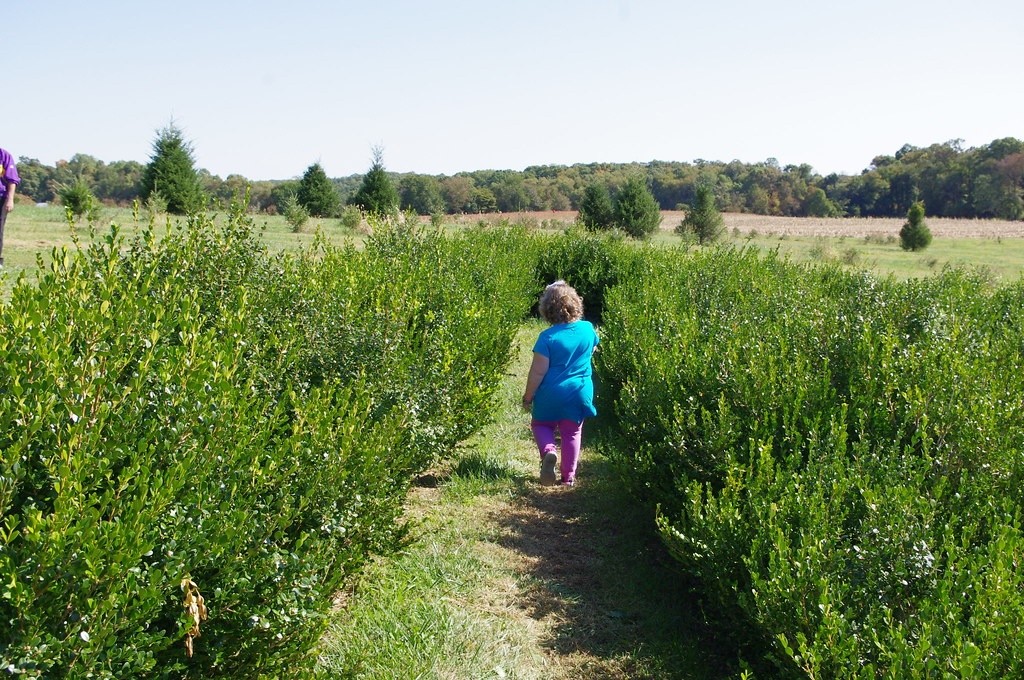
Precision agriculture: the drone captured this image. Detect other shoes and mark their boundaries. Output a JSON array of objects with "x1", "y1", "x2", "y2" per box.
[
  {"x1": 560, "y1": 477, "x2": 573, "y2": 487},
  {"x1": 541, "y1": 451, "x2": 556, "y2": 485}
]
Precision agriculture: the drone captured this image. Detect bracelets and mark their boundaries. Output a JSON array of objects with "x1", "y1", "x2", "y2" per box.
[{"x1": 522, "y1": 394, "x2": 531, "y2": 404}]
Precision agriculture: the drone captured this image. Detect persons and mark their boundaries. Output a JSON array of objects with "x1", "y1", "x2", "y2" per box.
[
  {"x1": 0, "y1": 147, "x2": 21, "y2": 264},
  {"x1": 521, "y1": 281, "x2": 600, "y2": 486}
]
